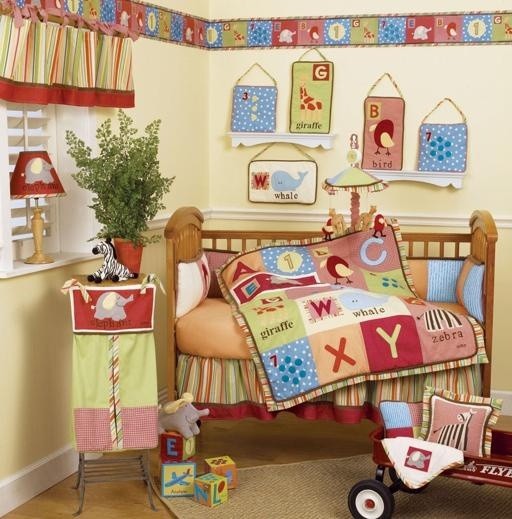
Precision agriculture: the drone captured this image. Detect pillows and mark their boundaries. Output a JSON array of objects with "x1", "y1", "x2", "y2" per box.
[
  {"x1": 454, "y1": 255, "x2": 485, "y2": 330},
  {"x1": 202, "y1": 248, "x2": 240, "y2": 298},
  {"x1": 407, "y1": 255, "x2": 466, "y2": 303},
  {"x1": 419, "y1": 383, "x2": 502, "y2": 457},
  {"x1": 378, "y1": 400, "x2": 423, "y2": 438},
  {"x1": 174, "y1": 250, "x2": 212, "y2": 319}
]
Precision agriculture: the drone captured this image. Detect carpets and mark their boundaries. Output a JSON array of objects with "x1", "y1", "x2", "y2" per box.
[{"x1": 145, "y1": 452, "x2": 511, "y2": 518}]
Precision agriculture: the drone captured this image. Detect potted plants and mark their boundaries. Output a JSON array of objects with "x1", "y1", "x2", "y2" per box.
[{"x1": 63, "y1": 107, "x2": 175, "y2": 275}]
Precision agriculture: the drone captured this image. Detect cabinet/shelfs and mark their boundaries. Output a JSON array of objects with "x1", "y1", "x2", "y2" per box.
[{"x1": 69, "y1": 273, "x2": 159, "y2": 515}]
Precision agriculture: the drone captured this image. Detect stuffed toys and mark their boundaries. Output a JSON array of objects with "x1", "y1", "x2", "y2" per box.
[
  {"x1": 156, "y1": 391, "x2": 213, "y2": 441},
  {"x1": 89, "y1": 238, "x2": 139, "y2": 283}
]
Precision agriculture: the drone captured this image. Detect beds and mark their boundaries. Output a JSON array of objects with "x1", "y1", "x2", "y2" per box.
[{"x1": 159, "y1": 205, "x2": 498, "y2": 424}]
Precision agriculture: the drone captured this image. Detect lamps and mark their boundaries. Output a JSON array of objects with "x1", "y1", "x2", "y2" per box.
[{"x1": 9, "y1": 151, "x2": 66, "y2": 264}]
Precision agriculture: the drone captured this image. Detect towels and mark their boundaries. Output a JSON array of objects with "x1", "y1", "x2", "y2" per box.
[{"x1": 381, "y1": 437, "x2": 465, "y2": 490}]
